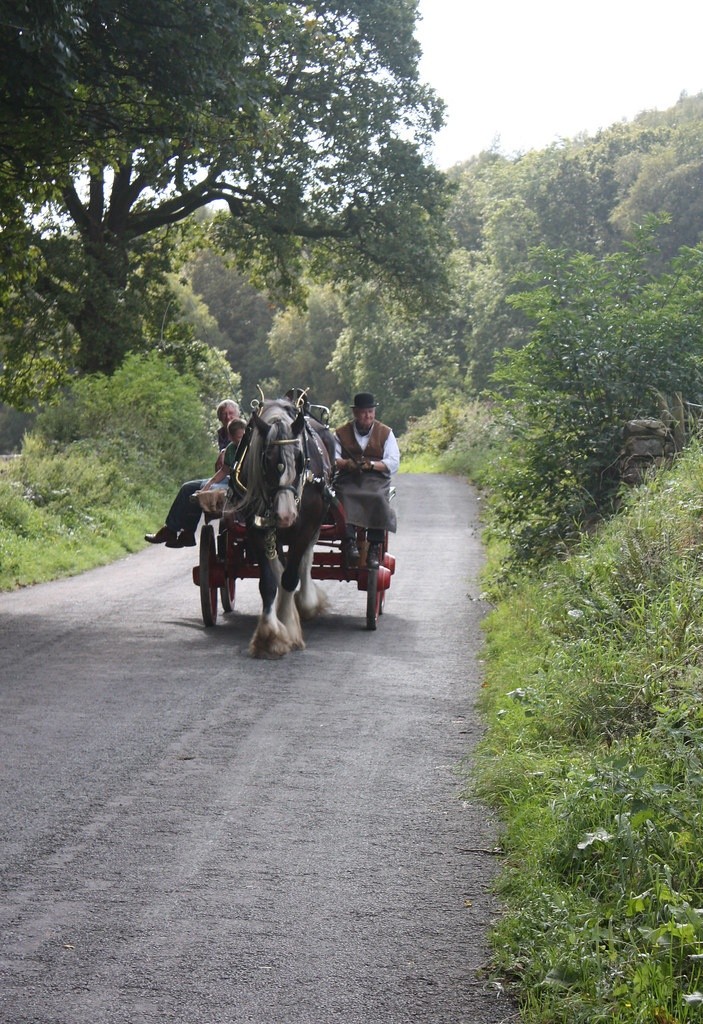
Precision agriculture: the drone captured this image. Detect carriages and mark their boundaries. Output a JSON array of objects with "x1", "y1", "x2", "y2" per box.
[{"x1": 191, "y1": 381, "x2": 397, "y2": 659}]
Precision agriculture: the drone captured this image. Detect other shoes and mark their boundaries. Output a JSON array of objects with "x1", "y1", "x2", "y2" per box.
[
  {"x1": 145, "y1": 526, "x2": 177, "y2": 544},
  {"x1": 165, "y1": 530, "x2": 196, "y2": 548},
  {"x1": 366, "y1": 547, "x2": 380, "y2": 568},
  {"x1": 340, "y1": 536, "x2": 360, "y2": 558}
]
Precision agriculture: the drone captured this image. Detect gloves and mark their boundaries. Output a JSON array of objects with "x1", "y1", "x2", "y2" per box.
[{"x1": 347, "y1": 458, "x2": 372, "y2": 474}]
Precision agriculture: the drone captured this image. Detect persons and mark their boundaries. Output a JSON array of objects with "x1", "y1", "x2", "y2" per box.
[
  {"x1": 145, "y1": 399, "x2": 247, "y2": 548},
  {"x1": 333, "y1": 392, "x2": 399, "y2": 570}
]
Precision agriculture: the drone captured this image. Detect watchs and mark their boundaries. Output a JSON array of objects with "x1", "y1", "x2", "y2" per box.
[{"x1": 370, "y1": 461, "x2": 375, "y2": 471}]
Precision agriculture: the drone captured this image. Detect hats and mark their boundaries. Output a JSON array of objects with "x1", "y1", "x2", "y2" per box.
[
  {"x1": 284, "y1": 387, "x2": 307, "y2": 408},
  {"x1": 350, "y1": 393, "x2": 379, "y2": 408}
]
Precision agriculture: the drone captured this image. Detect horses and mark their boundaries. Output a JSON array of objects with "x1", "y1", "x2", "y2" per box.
[{"x1": 222, "y1": 388, "x2": 339, "y2": 661}]
